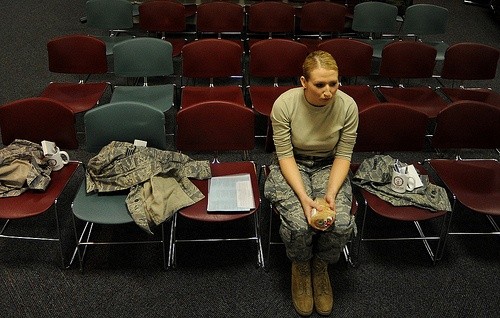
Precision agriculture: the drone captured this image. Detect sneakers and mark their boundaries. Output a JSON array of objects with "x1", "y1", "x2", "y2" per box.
[
  {"x1": 311, "y1": 261, "x2": 333, "y2": 316},
  {"x1": 290, "y1": 260, "x2": 313, "y2": 316}
]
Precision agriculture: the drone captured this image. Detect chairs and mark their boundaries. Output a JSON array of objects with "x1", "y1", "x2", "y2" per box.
[{"x1": 0, "y1": 0, "x2": 500, "y2": 269}]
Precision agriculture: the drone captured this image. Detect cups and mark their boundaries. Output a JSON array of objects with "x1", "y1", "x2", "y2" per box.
[
  {"x1": 391, "y1": 168, "x2": 415, "y2": 194},
  {"x1": 44, "y1": 146, "x2": 69, "y2": 171}
]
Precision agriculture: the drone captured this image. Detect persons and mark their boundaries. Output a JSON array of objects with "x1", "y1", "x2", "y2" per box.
[{"x1": 264, "y1": 51, "x2": 359, "y2": 315}]
